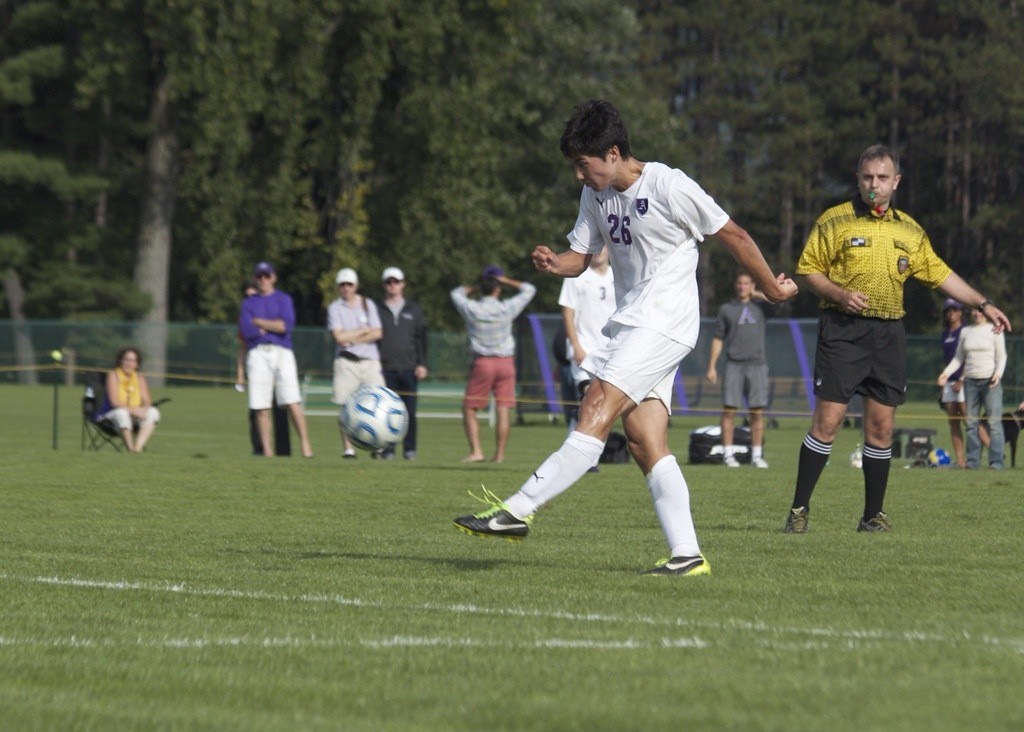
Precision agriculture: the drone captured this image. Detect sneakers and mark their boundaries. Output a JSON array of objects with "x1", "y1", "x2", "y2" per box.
[
  {"x1": 638, "y1": 550, "x2": 712, "y2": 580},
  {"x1": 452, "y1": 483, "x2": 535, "y2": 543},
  {"x1": 783, "y1": 504, "x2": 810, "y2": 534},
  {"x1": 856, "y1": 510, "x2": 895, "y2": 533}
]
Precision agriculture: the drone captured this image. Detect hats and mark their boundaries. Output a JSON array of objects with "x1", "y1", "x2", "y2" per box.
[
  {"x1": 255, "y1": 261, "x2": 272, "y2": 276},
  {"x1": 381, "y1": 266, "x2": 406, "y2": 281},
  {"x1": 242, "y1": 279, "x2": 256, "y2": 287},
  {"x1": 943, "y1": 297, "x2": 963, "y2": 312},
  {"x1": 480, "y1": 265, "x2": 504, "y2": 278},
  {"x1": 335, "y1": 267, "x2": 359, "y2": 286}
]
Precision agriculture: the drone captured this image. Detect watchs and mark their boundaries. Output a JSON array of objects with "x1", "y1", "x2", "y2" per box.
[{"x1": 977, "y1": 299, "x2": 994, "y2": 312}]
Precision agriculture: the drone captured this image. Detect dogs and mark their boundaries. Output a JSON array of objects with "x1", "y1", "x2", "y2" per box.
[{"x1": 980, "y1": 409, "x2": 1024, "y2": 467}]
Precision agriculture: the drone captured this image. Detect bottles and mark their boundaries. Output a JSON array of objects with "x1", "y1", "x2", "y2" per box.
[
  {"x1": 85, "y1": 388, "x2": 94, "y2": 398},
  {"x1": 853, "y1": 442, "x2": 863, "y2": 469}
]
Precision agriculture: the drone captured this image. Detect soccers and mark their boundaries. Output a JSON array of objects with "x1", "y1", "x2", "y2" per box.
[{"x1": 338, "y1": 385, "x2": 410, "y2": 454}]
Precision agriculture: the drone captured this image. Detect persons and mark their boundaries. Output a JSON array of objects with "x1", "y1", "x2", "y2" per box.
[
  {"x1": 453, "y1": 101, "x2": 798, "y2": 577},
  {"x1": 784, "y1": 146, "x2": 1012, "y2": 534},
  {"x1": 941, "y1": 298, "x2": 1006, "y2": 469},
  {"x1": 237, "y1": 242, "x2": 617, "y2": 475},
  {"x1": 707, "y1": 271, "x2": 777, "y2": 468},
  {"x1": 95, "y1": 346, "x2": 160, "y2": 453},
  {"x1": 938, "y1": 307, "x2": 1007, "y2": 469}
]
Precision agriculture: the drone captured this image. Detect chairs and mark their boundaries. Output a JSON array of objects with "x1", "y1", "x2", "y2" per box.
[{"x1": 80, "y1": 370, "x2": 138, "y2": 454}]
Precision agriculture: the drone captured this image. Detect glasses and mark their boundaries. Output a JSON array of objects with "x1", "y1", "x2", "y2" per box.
[
  {"x1": 339, "y1": 283, "x2": 353, "y2": 286},
  {"x1": 385, "y1": 280, "x2": 399, "y2": 284}
]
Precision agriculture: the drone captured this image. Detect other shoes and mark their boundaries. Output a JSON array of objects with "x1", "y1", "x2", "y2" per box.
[
  {"x1": 948, "y1": 454, "x2": 1006, "y2": 471},
  {"x1": 372, "y1": 450, "x2": 396, "y2": 460},
  {"x1": 750, "y1": 458, "x2": 768, "y2": 468},
  {"x1": 234, "y1": 384, "x2": 244, "y2": 392},
  {"x1": 462, "y1": 456, "x2": 503, "y2": 464},
  {"x1": 342, "y1": 449, "x2": 355, "y2": 457},
  {"x1": 404, "y1": 450, "x2": 416, "y2": 460},
  {"x1": 723, "y1": 456, "x2": 740, "y2": 468}
]
